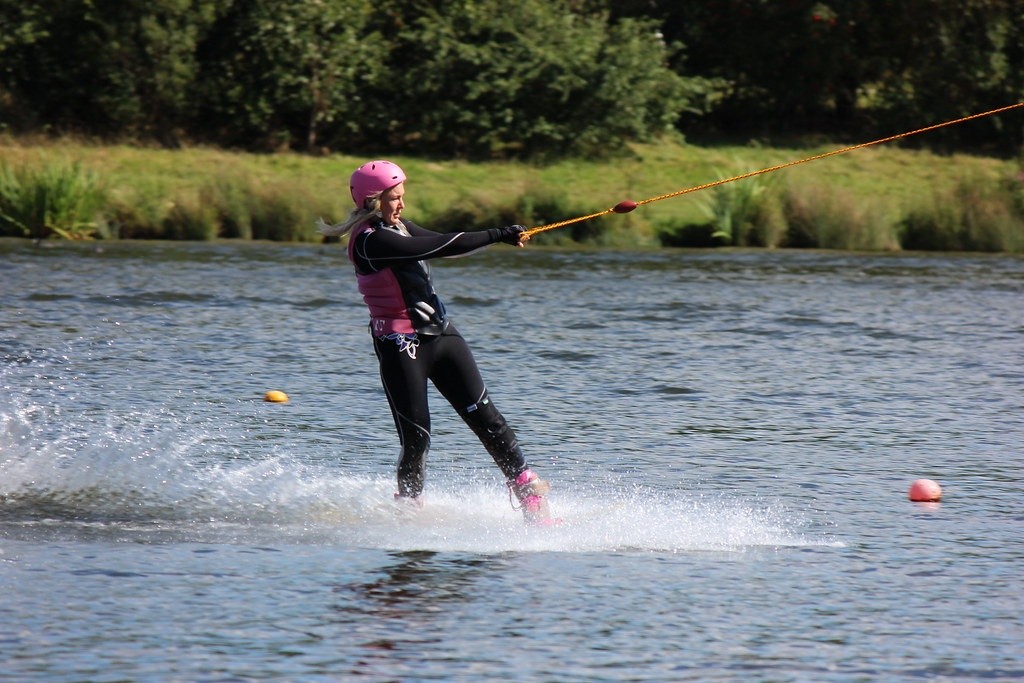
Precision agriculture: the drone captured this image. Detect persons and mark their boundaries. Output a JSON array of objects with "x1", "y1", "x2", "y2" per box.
[{"x1": 347, "y1": 160, "x2": 553, "y2": 529}]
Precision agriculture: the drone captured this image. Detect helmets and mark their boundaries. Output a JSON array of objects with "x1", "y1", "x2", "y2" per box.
[{"x1": 351, "y1": 160, "x2": 406, "y2": 209}]
